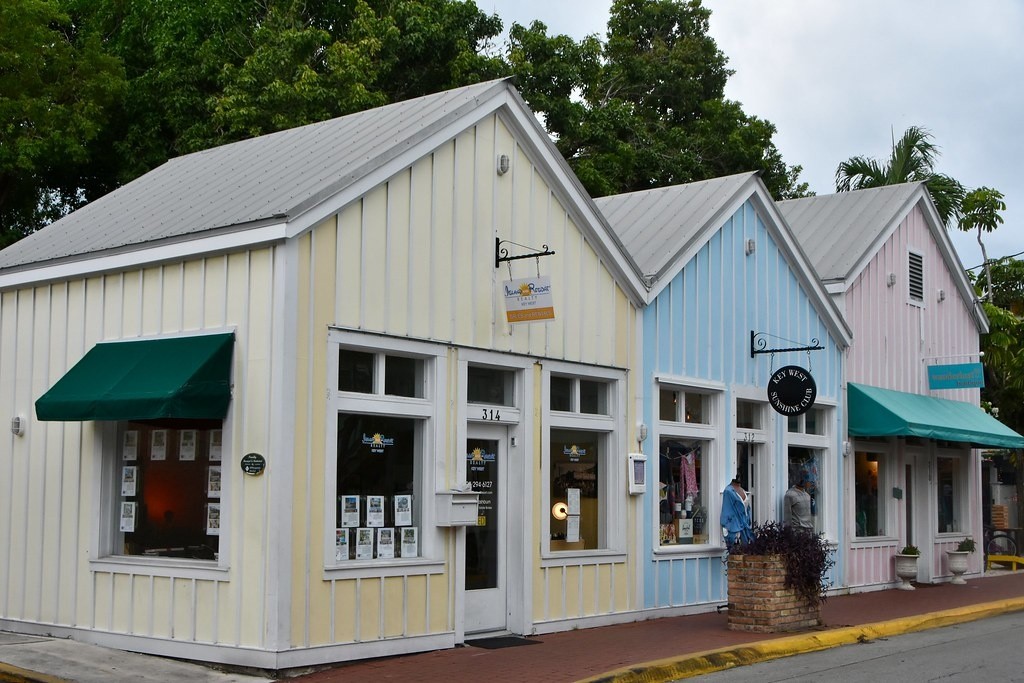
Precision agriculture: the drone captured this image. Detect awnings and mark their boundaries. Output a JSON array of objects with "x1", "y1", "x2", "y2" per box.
[
  {"x1": 35, "y1": 333, "x2": 234, "y2": 423},
  {"x1": 847, "y1": 382, "x2": 1024, "y2": 450}
]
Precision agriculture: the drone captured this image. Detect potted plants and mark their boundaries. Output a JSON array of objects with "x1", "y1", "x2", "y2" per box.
[
  {"x1": 896, "y1": 545, "x2": 922, "y2": 592},
  {"x1": 722, "y1": 520, "x2": 838, "y2": 635},
  {"x1": 944, "y1": 538, "x2": 978, "y2": 585}
]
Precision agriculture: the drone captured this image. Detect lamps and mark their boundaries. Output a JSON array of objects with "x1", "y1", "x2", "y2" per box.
[{"x1": 551, "y1": 501, "x2": 569, "y2": 521}]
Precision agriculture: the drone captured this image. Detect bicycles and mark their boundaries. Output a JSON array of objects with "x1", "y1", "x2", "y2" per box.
[{"x1": 984, "y1": 524, "x2": 1018, "y2": 565}]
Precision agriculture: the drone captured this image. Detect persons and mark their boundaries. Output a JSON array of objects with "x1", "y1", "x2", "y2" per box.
[
  {"x1": 721, "y1": 479, "x2": 755, "y2": 550},
  {"x1": 784, "y1": 481, "x2": 813, "y2": 537}
]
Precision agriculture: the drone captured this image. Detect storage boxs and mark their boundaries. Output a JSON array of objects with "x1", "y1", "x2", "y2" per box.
[{"x1": 675, "y1": 519, "x2": 693, "y2": 544}]
